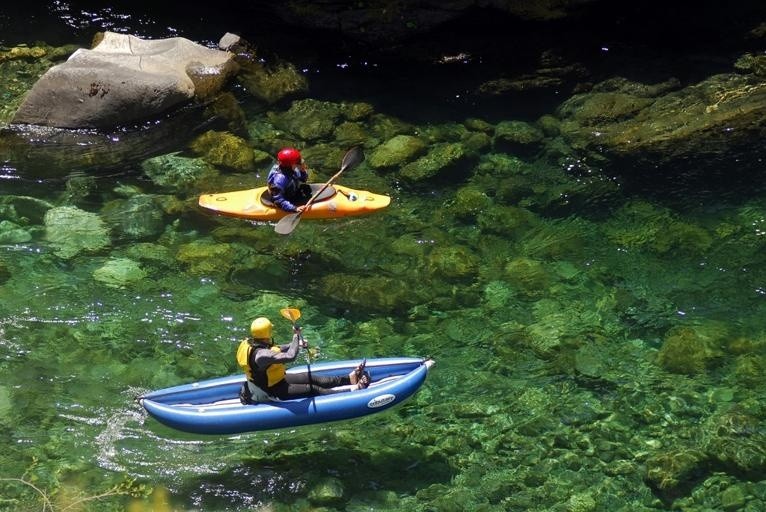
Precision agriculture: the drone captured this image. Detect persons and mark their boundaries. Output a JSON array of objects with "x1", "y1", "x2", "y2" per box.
[
  {"x1": 267, "y1": 149, "x2": 312, "y2": 212},
  {"x1": 235, "y1": 317, "x2": 370, "y2": 401}
]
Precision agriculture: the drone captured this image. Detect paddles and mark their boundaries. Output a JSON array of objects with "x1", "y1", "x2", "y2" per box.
[
  {"x1": 280, "y1": 309, "x2": 316, "y2": 360},
  {"x1": 274, "y1": 145, "x2": 365, "y2": 234}
]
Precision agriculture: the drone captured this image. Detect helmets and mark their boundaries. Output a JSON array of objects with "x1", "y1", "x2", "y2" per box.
[
  {"x1": 278, "y1": 149, "x2": 304, "y2": 167},
  {"x1": 250, "y1": 318, "x2": 275, "y2": 339}
]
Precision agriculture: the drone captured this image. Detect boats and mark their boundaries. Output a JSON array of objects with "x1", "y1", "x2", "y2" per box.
[
  {"x1": 135, "y1": 358, "x2": 438, "y2": 436},
  {"x1": 200, "y1": 183, "x2": 391, "y2": 220}
]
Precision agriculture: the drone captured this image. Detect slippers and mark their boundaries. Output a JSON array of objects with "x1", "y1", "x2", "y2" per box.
[{"x1": 354, "y1": 357, "x2": 371, "y2": 391}]
